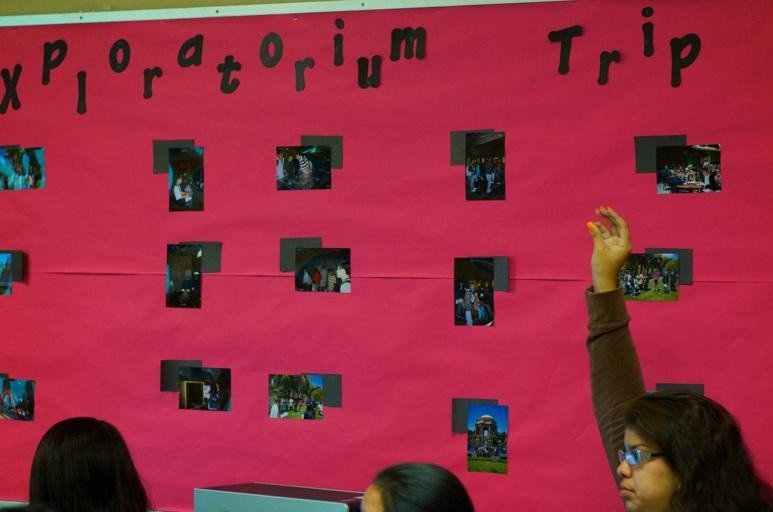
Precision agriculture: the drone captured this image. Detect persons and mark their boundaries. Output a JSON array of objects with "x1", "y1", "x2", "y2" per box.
[
  {"x1": 28, "y1": 413, "x2": 156, "y2": 510},
  {"x1": 188, "y1": 271, "x2": 200, "y2": 308},
  {"x1": 212, "y1": 381, "x2": 225, "y2": 407},
  {"x1": 578, "y1": 203, "x2": 773, "y2": 512},
  {"x1": 0, "y1": 379, "x2": 32, "y2": 420},
  {"x1": 168, "y1": 168, "x2": 205, "y2": 210},
  {"x1": 271, "y1": 394, "x2": 323, "y2": 420},
  {"x1": 454, "y1": 274, "x2": 494, "y2": 326},
  {"x1": 277, "y1": 148, "x2": 314, "y2": 185},
  {"x1": 355, "y1": 460, "x2": 477, "y2": 511},
  {"x1": 466, "y1": 153, "x2": 505, "y2": 199},
  {"x1": 622, "y1": 266, "x2": 678, "y2": 296},
  {"x1": 468, "y1": 434, "x2": 507, "y2": 464},
  {"x1": 658, "y1": 160, "x2": 722, "y2": 194},
  {"x1": 180, "y1": 276, "x2": 191, "y2": 297},
  {"x1": 300, "y1": 257, "x2": 351, "y2": 293}
]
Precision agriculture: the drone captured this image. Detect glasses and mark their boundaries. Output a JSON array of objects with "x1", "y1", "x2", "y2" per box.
[{"x1": 616, "y1": 447, "x2": 665, "y2": 466}]
